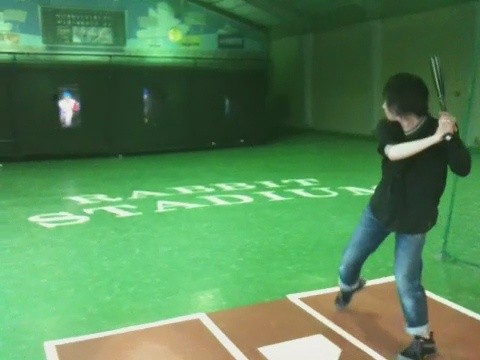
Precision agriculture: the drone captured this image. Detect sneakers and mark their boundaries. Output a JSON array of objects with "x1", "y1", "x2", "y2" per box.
[
  {"x1": 334, "y1": 279, "x2": 365, "y2": 308},
  {"x1": 396, "y1": 333, "x2": 435, "y2": 360}
]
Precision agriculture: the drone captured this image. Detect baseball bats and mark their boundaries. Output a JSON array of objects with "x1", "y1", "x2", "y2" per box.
[{"x1": 428, "y1": 55, "x2": 454, "y2": 141}]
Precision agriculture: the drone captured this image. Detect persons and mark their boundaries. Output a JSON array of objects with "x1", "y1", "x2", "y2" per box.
[{"x1": 335, "y1": 70, "x2": 473, "y2": 359}]
